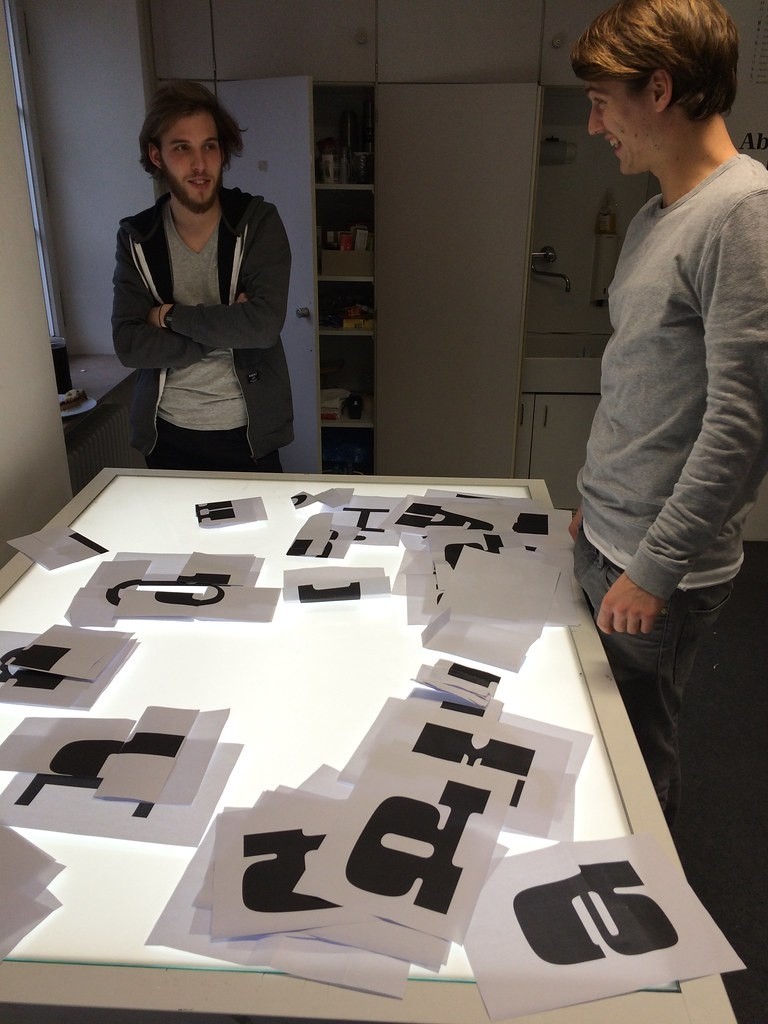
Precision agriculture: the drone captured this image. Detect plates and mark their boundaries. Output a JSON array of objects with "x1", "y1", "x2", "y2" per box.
[{"x1": 57, "y1": 393, "x2": 97, "y2": 418}]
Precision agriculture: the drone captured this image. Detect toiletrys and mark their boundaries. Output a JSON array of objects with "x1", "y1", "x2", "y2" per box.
[{"x1": 596, "y1": 199, "x2": 622, "y2": 235}]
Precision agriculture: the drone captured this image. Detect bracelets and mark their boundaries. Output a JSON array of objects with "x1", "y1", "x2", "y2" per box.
[{"x1": 159, "y1": 303, "x2": 165, "y2": 328}]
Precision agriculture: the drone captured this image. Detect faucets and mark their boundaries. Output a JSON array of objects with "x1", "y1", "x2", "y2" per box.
[{"x1": 530, "y1": 250, "x2": 572, "y2": 294}]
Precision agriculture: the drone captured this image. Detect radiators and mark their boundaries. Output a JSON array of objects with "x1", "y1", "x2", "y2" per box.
[{"x1": 65, "y1": 402, "x2": 136, "y2": 499}]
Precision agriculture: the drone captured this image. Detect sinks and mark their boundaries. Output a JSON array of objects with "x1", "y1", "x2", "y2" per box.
[{"x1": 521, "y1": 329, "x2": 612, "y2": 396}]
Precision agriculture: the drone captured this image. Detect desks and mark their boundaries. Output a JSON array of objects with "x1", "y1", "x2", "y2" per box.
[{"x1": 0, "y1": 467, "x2": 742, "y2": 1024}]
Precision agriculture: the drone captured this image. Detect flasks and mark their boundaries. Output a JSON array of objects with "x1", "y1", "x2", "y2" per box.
[
  {"x1": 341, "y1": 109, "x2": 355, "y2": 185},
  {"x1": 361, "y1": 101, "x2": 375, "y2": 151}
]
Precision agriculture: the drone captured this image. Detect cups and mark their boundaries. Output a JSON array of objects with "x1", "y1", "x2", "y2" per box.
[
  {"x1": 51, "y1": 336, "x2": 72, "y2": 395},
  {"x1": 352, "y1": 152, "x2": 372, "y2": 184},
  {"x1": 321, "y1": 154, "x2": 342, "y2": 184}
]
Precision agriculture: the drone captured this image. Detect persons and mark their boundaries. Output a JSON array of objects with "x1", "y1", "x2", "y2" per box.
[
  {"x1": 568, "y1": 0, "x2": 768, "y2": 836},
  {"x1": 111, "y1": 80, "x2": 300, "y2": 474}
]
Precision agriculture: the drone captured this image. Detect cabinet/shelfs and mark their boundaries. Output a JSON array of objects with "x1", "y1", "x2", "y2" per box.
[
  {"x1": 212, "y1": 2, "x2": 375, "y2": 81},
  {"x1": 370, "y1": 0, "x2": 543, "y2": 81},
  {"x1": 540, "y1": 0, "x2": 608, "y2": 84},
  {"x1": 215, "y1": 77, "x2": 542, "y2": 473},
  {"x1": 516, "y1": 353, "x2": 607, "y2": 519}
]
